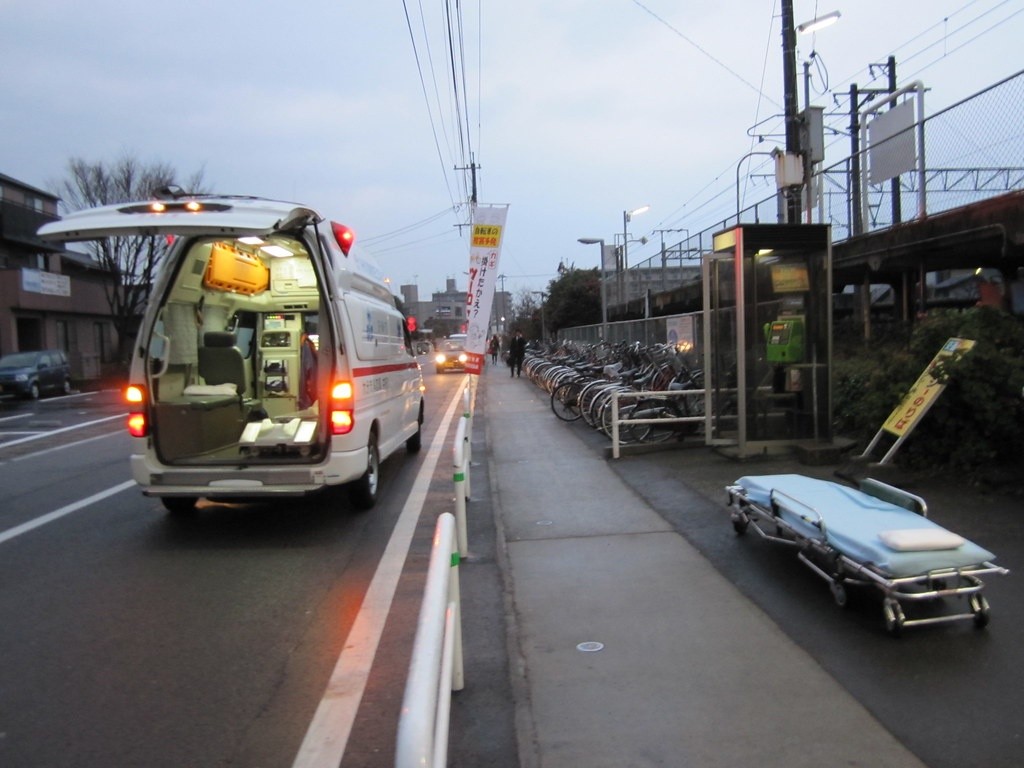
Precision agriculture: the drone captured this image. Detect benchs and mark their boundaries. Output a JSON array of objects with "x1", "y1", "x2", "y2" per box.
[{"x1": 159, "y1": 395, "x2": 239, "y2": 453}]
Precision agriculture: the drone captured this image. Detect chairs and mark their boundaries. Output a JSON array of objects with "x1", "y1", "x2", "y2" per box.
[{"x1": 197, "y1": 328, "x2": 260, "y2": 411}]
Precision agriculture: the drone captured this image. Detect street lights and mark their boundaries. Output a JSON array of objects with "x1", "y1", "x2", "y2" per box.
[
  {"x1": 620, "y1": 206, "x2": 651, "y2": 322},
  {"x1": 531, "y1": 291, "x2": 547, "y2": 344},
  {"x1": 578, "y1": 236, "x2": 610, "y2": 348},
  {"x1": 614, "y1": 238, "x2": 649, "y2": 324}
]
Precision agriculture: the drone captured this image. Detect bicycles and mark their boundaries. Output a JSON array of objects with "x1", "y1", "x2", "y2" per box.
[{"x1": 500, "y1": 338, "x2": 730, "y2": 445}]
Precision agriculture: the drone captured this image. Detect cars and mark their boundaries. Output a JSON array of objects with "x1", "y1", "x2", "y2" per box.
[
  {"x1": 448, "y1": 334, "x2": 468, "y2": 351},
  {"x1": 0, "y1": 348, "x2": 75, "y2": 400},
  {"x1": 434, "y1": 338, "x2": 468, "y2": 374}
]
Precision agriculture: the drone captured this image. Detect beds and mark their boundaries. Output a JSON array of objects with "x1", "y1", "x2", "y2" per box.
[{"x1": 728, "y1": 474, "x2": 1007, "y2": 641}]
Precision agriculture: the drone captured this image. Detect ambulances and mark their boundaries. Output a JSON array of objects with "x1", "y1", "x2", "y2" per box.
[{"x1": 33, "y1": 182, "x2": 425, "y2": 516}]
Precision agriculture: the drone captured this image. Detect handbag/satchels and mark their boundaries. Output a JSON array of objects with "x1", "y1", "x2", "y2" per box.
[
  {"x1": 506, "y1": 355, "x2": 511, "y2": 367},
  {"x1": 487, "y1": 348, "x2": 492, "y2": 354}
]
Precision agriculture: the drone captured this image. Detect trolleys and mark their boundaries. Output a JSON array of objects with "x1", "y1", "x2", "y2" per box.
[{"x1": 725, "y1": 467, "x2": 1012, "y2": 639}]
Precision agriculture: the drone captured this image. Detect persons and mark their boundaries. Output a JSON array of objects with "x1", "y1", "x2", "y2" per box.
[
  {"x1": 488, "y1": 332, "x2": 502, "y2": 365},
  {"x1": 508, "y1": 329, "x2": 526, "y2": 377}
]
